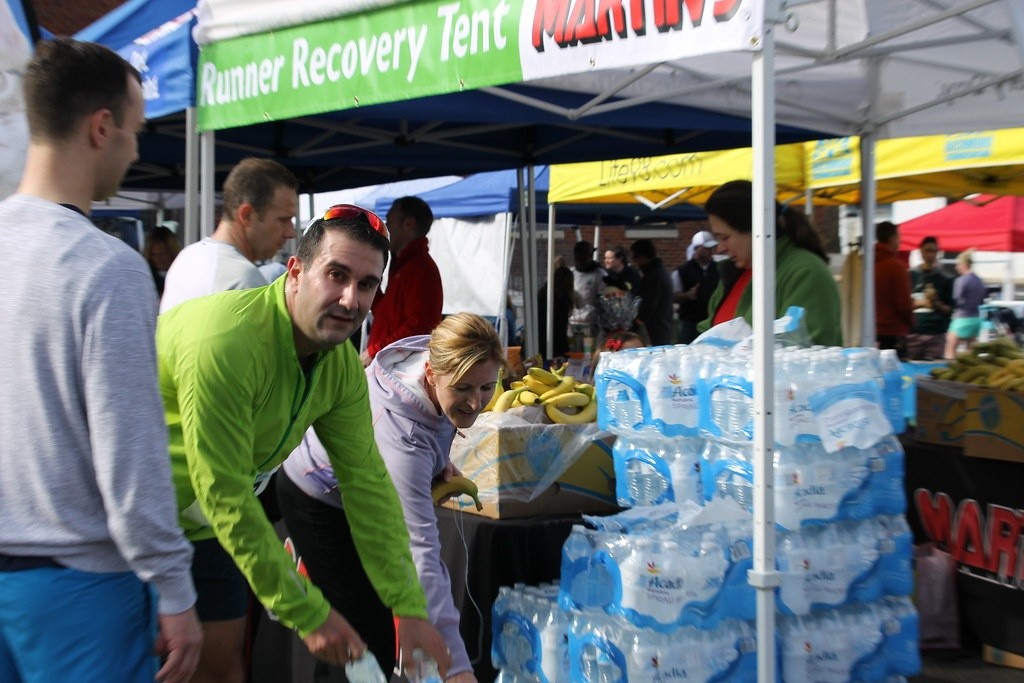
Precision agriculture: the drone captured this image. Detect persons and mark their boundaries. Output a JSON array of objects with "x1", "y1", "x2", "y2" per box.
[
  {"x1": 358, "y1": 197, "x2": 443, "y2": 367},
  {"x1": 910, "y1": 236, "x2": 952, "y2": 335},
  {"x1": 1, "y1": 37, "x2": 205, "y2": 683},
  {"x1": 276, "y1": 314, "x2": 507, "y2": 683},
  {"x1": 602, "y1": 245, "x2": 640, "y2": 296},
  {"x1": 155, "y1": 204, "x2": 452, "y2": 683},
  {"x1": 671, "y1": 231, "x2": 719, "y2": 344},
  {"x1": 567, "y1": 241, "x2": 609, "y2": 338},
  {"x1": 943, "y1": 252, "x2": 986, "y2": 360},
  {"x1": 875, "y1": 221, "x2": 915, "y2": 360},
  {"x1": 694, "y1": 179, "x2": 844, "y2": 347},
  {"x1": 145, "y1": 226, "x2": 180, "y2": 295},
  {"x1": 631, "y1": 239, "x2": 673, "y2": 346},
  {"x1": 539, "y1": 266, "x2": 573, "y2": 355},
  {"x1": 160, "y1": 158, "x2": 297, "y2": 316}
]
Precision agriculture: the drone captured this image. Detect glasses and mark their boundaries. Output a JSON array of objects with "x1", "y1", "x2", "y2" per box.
[{"x1": 301, "y1": 204, "x2": 390, "y2": 242}]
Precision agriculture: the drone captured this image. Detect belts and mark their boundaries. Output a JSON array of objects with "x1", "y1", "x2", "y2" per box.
[{"x1": 1, "y1": 555, "x2": 65, "y2": 572}]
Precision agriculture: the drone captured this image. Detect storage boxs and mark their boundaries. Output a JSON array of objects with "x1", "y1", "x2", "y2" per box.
[
  {"x1": 915, "y1": 377, "x2": 1024, "y2": 463},
  {"x1": 440, "y1": 422, "x2": 618, "y2": 520}
]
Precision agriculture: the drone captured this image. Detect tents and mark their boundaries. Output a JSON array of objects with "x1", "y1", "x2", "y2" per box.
[{"x1": 0, "y1": 1, "x2": 1024, "y2": 682}]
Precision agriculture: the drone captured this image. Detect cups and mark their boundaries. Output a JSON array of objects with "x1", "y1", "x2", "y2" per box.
[{"x1": 582, "y1": 338, "x2": 594, "y2": 355}]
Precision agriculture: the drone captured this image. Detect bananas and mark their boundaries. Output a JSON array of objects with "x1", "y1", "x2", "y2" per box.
[
  {"x1": 431, "y1": 476, "x2": 483, "y2": 511},
  {"x1": 480, "y1": 362, "x2": 596, "y2": 424},
  {"x1": 928, "y1": 336, "x2": 1024, "y2": 394}
]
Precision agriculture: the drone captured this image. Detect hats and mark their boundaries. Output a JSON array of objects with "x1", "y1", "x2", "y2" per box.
[{"x1": 692, "y1": 231, "x2": 719, "y2": 248}]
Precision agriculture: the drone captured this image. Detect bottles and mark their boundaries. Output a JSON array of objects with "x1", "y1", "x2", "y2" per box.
[
  {"x1": 557, "y1": 512, "x2": 914, "y2": 635},
  {"x1": 573, "y1": 332, "x2": 584, "y2": 352},
  {"x1": 613, "y1": 435, "x2": 905, "y2": 534},
  {"x1": 594, "y1": 344, "x2": 906, "y2": 454},
  {"x1": 569, "y1": 595, "x2": 920, "y2": 683},
  {"x1": 491, "y1": 579, "x2": 569, "y2": 683},
  {"x1": 420, "y1": 661, "x2": 444, "y2": 683},
  {"x1": 345, "y1": 649, "x2": 388, "y2": 683}
]
